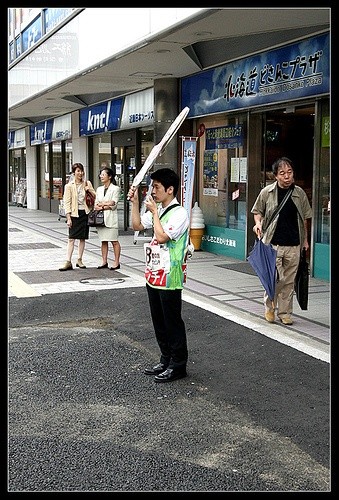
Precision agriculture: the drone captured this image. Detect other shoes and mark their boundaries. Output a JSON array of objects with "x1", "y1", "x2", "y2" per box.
[
  {"x1": 279, "y1": 316, "x2": 293, "y2": 325},
  {"x1": 110, "y1": 264, "x2": 120, "y2": 270},
  {"x1": 265, "y1": 309, "x2": 275, "y2": 322},
  {"x1": 97, "y1": 262, "x2": 108, "y2": 269},
  {"x1": 59, "y1": 264, "x2": 72, "y2": 271},
  {"x1": 76, "y1": 263, "x2": 86, "y2": 269}
]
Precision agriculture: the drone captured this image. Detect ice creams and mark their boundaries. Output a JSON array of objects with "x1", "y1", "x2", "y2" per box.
[{"x1": 190, "y1": 202, "x2": 205, "y2": 250}]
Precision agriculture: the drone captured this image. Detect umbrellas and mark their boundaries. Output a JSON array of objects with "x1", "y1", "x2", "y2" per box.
[{"x1": 246, "y1": 230, "x2": 278, "y2": 310}]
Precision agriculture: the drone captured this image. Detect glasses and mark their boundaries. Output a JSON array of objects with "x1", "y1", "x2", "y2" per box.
[{"x1": 100, "y1": 174, "x2": 109, "y2": 177}]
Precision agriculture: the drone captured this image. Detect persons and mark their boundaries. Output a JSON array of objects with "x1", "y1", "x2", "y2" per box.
[
  {"x1": 59, "y1": 164, "x2": 95, "y2": 272},
  {"x1": 251, "y1": 156, "x2": 314, "y2": 325},
  {"x1": 93, "y1": 168, "x2": 122, "y2": 270},
  {"x1": 127, "y1": 169, "x2": 189, "y2": 382}
]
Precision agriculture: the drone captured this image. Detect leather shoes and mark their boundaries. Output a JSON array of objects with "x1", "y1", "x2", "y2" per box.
[
  {"x1": 144, "y1": 363, "x2": 167, "y2": 375},
  {"x1": 154, "y1": 368, "x2": 186, "y2": 382}
]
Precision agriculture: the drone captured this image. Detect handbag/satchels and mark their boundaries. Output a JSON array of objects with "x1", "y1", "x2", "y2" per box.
[
  {"x1": 88, "y1": 208, "x2": 106, "y2": 227},
  {"x1": 85, "y1": 180, "x2": 95, "y2": 208},
  {"x1": 293, "y1": 247, "x2": 308, "y2": 310}
]
